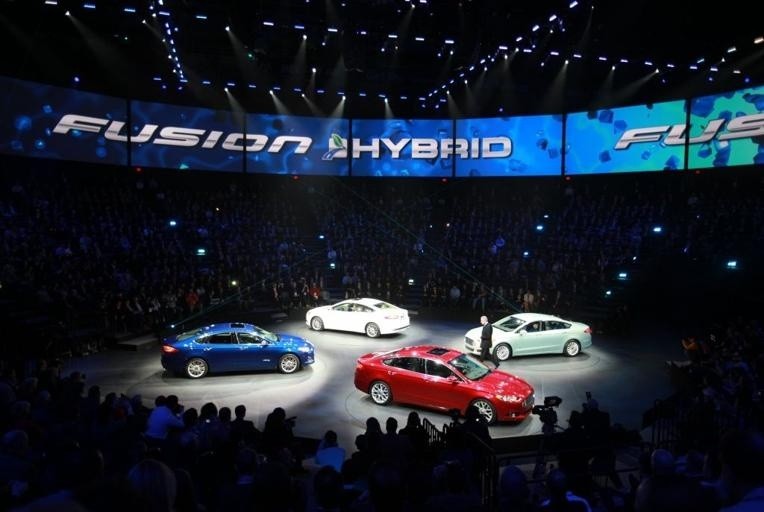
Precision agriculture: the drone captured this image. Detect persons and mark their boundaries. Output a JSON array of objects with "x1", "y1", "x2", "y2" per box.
[{"x1": 0, "y1": 176, "x2": 764, "y2": 512}]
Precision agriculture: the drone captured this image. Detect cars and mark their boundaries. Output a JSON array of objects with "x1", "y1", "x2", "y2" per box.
[
  {"x1": 464, "y1": 312, "x2": 593, "y2": 362},
  {"x1": 354, "y1": 345, "x2": 535, "y2": 425},
  {"x1": 306, "y1": 298, "x2": 411, "y2": 338},
  {"x1": 161, "y1": 323, "x2": 315, "y2": 380}
]
